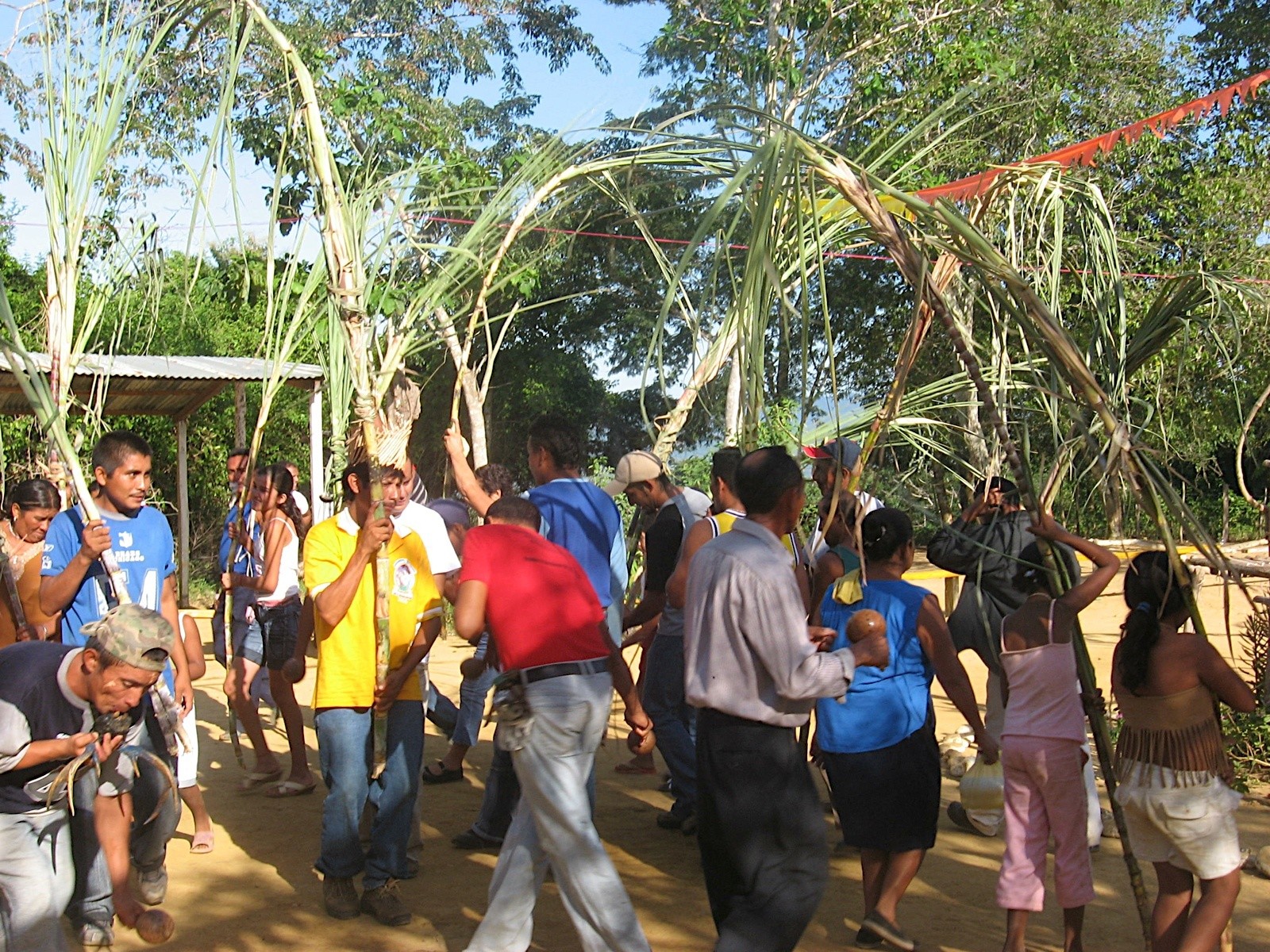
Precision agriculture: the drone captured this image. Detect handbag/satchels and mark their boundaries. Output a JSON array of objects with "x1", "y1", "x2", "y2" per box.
[{"x1": 958, "y1": 756, "x2": 1007, "y2": 811}]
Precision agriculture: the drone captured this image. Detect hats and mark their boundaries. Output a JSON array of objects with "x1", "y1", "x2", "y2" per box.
[
  {"x1": 79, "y1": 604, "x2": 175, "y2": 675},
  {"x1": 603, "y1": 449, "x2": 665, "y2": 495},
  {"x1": 802, "y1": 437, "x2": 861, "y2": 470}
]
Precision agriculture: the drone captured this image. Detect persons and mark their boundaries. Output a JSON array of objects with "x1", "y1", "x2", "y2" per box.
[
  {"x1": 997, "y1": 498, "x2": 1120, "y2": 952},
  {"x1": 0, "y1": 604, "x2": 174, "y2": 952},
  {"x1": 0, "y1": 447, "x2": 884, "y2": 880},
  {"x1": 810, "y1": 509, "x2": 999, "y2": 950},
  {"x1": 451, "y1": 414, "x2": 629, "y2": 851},
  {"x1": 926, "y1": 477, "x2": 1104, "y2": 853},
  {"x1": 454, "y1": 496, "x2": 654, "y2": 952},
  {"x1": 221, "y1": 464, "x2": 317, "y2": 798},
  {"x1": 683, "y1": 446, "x2": 889, "y2": 952},
  {"x1": 40, "y1": 432, "x2": 194, "y2": 906},
  {"x1": 304, "y1": 457, "x2": 443, "y2": 926},
  {"x1": 1111, "y1": 551, "x2": 1258, "y2": 952}
]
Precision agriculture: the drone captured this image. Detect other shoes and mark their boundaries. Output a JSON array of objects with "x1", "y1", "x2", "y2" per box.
[
  {"x1": 405, "y1": 827, "x2": 425, "y2": 850},
  {"x1": 422, "y1": 760, "x2": 464, "y2": 784},
  {"x1": 660, "y1": 777, "x2": 672, "y2": 790},
  {"x1": 1089, "y1": 845, "x2": 1101, "y2": 852},
  {"x1": 946, "y1": 800, "x2": 982, "y2": 836},
  {"x1": 404, "y1": 854, "x2": 419, "y2": 877},
  {"x1": 456, "y1": 829, "x2": 503, "y2": 849},
  {"x1": 655, "y1": 810, "x2": 688, "y2": 829},
  {"x1": 135, "y1": 865, "x2": 168, "y2": 904},
  {"x1": 681, "y1": 816, "x2": 697, "y2": 836},
  {"x1": 219, "y1": 729, "x2": 249, "y2": 739},
  {"x1": 268, "y1": 707, "x2": 282, "y2": 725},
  {"x1": 76, "y1": 920, "x2": 115, "y2": 946},
  {"x1": 853, "y1": 910, "x2": 920, "y2": 952}
]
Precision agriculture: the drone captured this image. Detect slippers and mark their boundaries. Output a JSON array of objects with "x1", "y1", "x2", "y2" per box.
[
  {"x1": 267, "y1": 780, "x2": 317, "y2": 797},
  {"x1": 238, "y1": 768, "x2": 282, "y2": 789},
  {"x1": 190, "y1": 814, "x2": 214, "y2": 852},
  {"x1": 614, "y1": 759, "x2": 656, "y2": 773}
]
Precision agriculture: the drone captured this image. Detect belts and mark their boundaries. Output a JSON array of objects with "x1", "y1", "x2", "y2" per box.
[{"x1": 499, "y1": 658, "x2": 613, "y2": 690}]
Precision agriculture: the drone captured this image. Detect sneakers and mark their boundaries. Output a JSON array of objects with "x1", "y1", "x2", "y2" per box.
[
  {"x1": 362, "y1": 878, "x2": 412, "y2": 926},
  {"x1": 322, "y1": 873, "x2": 362, "y2": 919}
]
